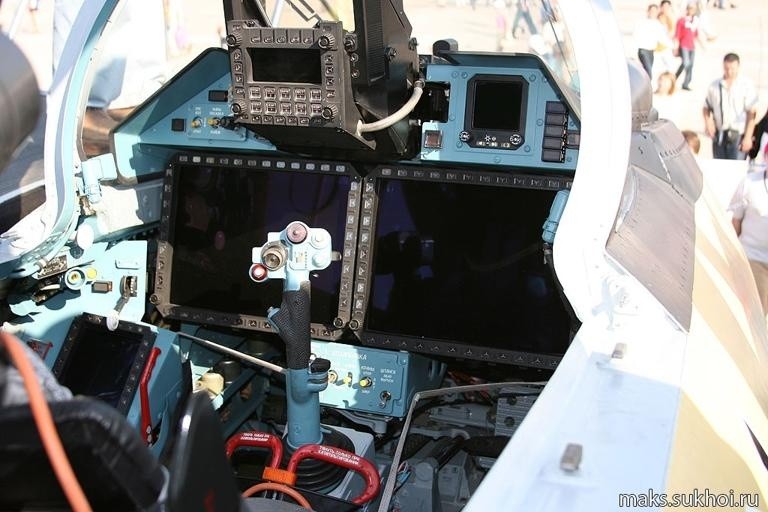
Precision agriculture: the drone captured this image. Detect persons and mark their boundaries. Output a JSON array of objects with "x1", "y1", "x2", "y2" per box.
[
  {"x1": 638, "y1": 1, "x2": 709, "y2": 91},
  {"x1": 653, "y1": 53, "x2": 768, "y2": 317}
]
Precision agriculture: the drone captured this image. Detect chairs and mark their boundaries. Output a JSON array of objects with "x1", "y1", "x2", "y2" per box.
[{"x1": 3, "y1": 397, "x2": 171, "y2": 510}]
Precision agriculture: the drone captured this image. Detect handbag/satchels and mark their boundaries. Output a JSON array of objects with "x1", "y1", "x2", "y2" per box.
[{"x1": 722, "y1": 129, "x2": 739, "y2": 148}]
